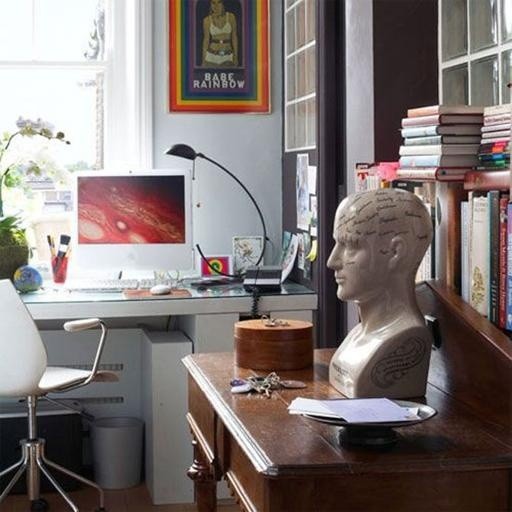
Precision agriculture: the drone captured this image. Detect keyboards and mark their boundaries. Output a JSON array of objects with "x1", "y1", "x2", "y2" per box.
[{"x1": 65, "y1": 278, "x2": 177, "y2": 292}]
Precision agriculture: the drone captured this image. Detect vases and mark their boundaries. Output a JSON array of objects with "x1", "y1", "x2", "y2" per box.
[{"x1": 1, "y1": 245, "x2": 29, "y2": 280}]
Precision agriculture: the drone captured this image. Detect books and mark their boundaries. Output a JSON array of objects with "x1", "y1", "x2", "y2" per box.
[{"x1": 380, "y1": 100, "x2": 512, "y2": 333}]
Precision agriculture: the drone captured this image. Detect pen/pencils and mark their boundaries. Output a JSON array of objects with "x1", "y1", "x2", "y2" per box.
[{"x1": 119, "y1": 271, "x2": 123, "y2": 279}]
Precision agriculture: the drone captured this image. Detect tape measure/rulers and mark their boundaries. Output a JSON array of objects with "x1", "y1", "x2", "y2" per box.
[{"x1": 14, "y1": 266, "x2": 42, "y2": 292}]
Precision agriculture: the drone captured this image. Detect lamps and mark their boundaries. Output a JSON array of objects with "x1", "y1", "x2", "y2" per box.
[{"x1": 165, "y1": 144, "x2": 267, "y2": 278}]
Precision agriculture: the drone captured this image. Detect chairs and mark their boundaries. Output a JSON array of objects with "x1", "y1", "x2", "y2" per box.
[{"x1": 0, "y1": 277, "x2": 108, "y2": 511}]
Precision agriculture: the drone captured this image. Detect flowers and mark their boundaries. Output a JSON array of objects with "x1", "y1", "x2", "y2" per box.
[{"x1": 0, "y1": 115, "x2": 72, "y2": 257}]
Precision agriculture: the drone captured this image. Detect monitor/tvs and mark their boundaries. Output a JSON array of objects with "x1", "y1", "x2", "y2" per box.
[{"x1": 71, "y1": 169, "x2": 193, "y2": 278}]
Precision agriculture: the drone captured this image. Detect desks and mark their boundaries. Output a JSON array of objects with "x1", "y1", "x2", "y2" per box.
[
  {"x1": 20, "y1": 275, "x2": 320, "y2": 503},
  {"x1": 180, "y1": 277, "x2": 511, "y2": 511}
]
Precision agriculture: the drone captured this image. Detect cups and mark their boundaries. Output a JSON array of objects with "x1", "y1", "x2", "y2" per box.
[{"x1": 52, "y1": 256, "x2": 68, "y2": 283}]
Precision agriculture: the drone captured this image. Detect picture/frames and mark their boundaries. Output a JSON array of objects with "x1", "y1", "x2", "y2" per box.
[
  {"x1": 164, "y1": 0, "x2": 271, "y2": 115},
  {"x1": 231, "y1": 235, "x2": 264, "y2": 275}
]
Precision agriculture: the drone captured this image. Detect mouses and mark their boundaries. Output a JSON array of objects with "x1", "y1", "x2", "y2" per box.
[{"x1": 150, "y1": 284, "x2": 170, "y2": 294}]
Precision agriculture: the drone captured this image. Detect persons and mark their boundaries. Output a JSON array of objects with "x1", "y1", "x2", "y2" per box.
[
  {"x1": 326, "y1": 187, "x2": 434, "y2": 400},
  {"x1": 201, "y1": 0, "x2": 239, "y2": 66}
]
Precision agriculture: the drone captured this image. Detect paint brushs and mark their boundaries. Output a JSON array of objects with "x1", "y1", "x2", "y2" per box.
[{"x1": 47, "y1": 235, "x2": 71, "y2": 272}]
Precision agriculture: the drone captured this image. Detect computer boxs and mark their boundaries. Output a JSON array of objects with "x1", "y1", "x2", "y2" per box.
[{"x1": 0, "y1": 399, "x2": 85, "y2": 495}]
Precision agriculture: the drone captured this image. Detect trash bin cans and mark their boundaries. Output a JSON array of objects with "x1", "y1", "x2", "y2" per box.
[{"x1": 88, "y1": 416, "x2": 145, "y2": 490}]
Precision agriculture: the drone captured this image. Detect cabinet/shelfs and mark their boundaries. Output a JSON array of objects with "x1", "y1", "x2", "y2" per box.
[
  {"x1": 282, "y1": 2, "x2": 317, "y2": 154},
  {"x1": 436, "y1": 1, "x2": 510, "y2": 104}
]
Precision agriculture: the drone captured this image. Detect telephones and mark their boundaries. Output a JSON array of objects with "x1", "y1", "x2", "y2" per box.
[{"x1": 243, "y1": 265, "x2": 281, "y2": 292}]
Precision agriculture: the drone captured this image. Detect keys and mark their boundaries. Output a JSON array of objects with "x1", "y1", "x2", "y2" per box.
[{"x1": 229, "y1": 372, "x2": 305, "y2": 399}]
